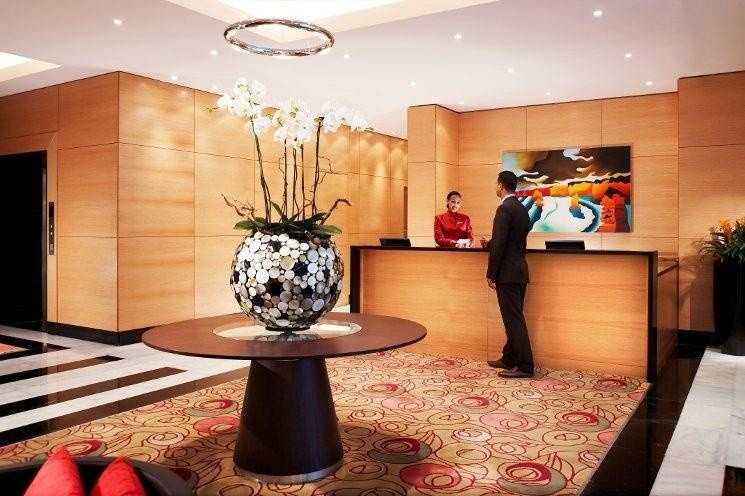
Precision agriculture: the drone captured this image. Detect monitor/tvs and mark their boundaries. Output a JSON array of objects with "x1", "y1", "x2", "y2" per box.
[
  {"x1": 545, "y1": 240, "x2": 585, "y2": 250},
  {"x1": 380, "y1": 238, "x2": 411, "y2": 246}
]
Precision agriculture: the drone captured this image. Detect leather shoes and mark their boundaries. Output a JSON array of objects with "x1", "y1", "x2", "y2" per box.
[
  {"x1": 487, "y1": 359, "x2": 511, "y2": 369},
  {"x1": 498, "y1": 366, "x2": 532, "y2": 378}
]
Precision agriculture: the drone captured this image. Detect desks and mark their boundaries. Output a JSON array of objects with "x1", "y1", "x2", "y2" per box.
[{"x1": 140, "y1": 313, "x2": 428, "y2": 486}]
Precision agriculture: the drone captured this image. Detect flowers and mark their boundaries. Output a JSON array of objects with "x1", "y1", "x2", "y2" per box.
[
  {"x1": 695, "y1": 215, "x2": 745, "y2": 265},
  {"x1": 202, "y1": 77, "x2": 374, "y2": 241}
]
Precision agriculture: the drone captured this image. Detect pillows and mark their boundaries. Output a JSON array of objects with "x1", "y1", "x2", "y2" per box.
[{"x1": 21, "y1": 445, "x2": 146, "y2": 496}]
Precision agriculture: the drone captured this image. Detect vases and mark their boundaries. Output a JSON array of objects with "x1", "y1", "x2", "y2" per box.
[
  {"x1": 712, "y1": 259, "x2": 745, "y2": 356},
  {"x1": 227, "y1": 230, "x2": 345, "y2": 331}
]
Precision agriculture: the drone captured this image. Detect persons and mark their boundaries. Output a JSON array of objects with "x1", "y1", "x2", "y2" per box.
[
  {"x1": 480, "y1": 171, "x2": 534, "y2": 379},
  {"x1": 435, "y1": 192, "x2": 475, "y2": 248}
]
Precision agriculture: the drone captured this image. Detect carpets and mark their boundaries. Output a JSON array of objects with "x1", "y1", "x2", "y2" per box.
[{"x1": 0, "y1": 348, "x2": 657, "y2": 496}]
